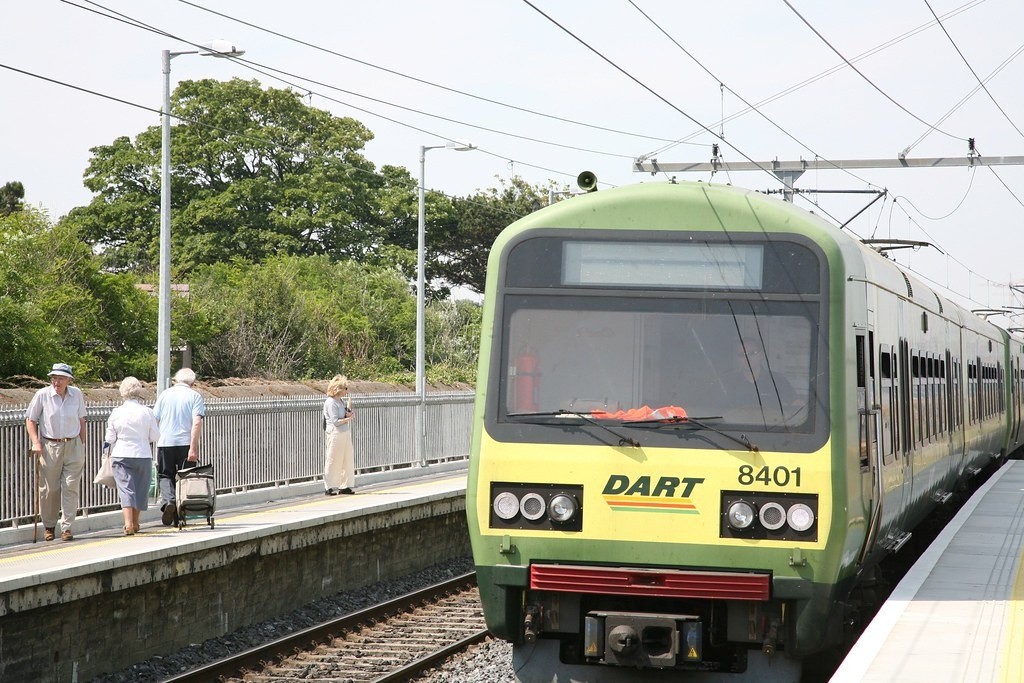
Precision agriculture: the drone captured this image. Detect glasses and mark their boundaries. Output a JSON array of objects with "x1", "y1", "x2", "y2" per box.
[{"x1": 736, "y1": 350, "x2": 761, "y2": 357}]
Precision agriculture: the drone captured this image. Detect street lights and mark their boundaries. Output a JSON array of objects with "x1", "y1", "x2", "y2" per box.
[
  {"x1": 159, "y1": 44, "x2": 242, "y2": 395},
  {"x1": 415, "y1": 143, "x2": 477, "y2": 394}
]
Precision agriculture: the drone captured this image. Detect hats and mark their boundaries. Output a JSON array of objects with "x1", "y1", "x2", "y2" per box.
[{"x1": 47, "y1": 363, "x2": 75, "y2": 381}]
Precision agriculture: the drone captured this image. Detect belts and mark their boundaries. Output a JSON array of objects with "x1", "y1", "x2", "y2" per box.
[{"x1": 42, "y1": 437, "x2": 72, "y2": 442}]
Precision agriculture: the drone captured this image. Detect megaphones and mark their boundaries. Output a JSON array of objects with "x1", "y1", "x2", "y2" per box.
[{"x1": 577, "y1": 171, "x2": 598, "y2": 191}]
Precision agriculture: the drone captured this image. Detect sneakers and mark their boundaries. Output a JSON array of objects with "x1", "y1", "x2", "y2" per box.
[{"x1": 162, "y1": 503, "x2": 175, "y2": 525}]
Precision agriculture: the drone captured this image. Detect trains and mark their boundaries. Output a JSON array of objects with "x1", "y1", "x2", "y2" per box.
[{"x1": 464, "y1": 184, "x2": 1024, "y2": 671}]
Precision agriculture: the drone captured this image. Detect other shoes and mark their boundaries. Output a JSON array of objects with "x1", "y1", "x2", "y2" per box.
[
  {"x1": 325, "y1": 488, "x2": 337, "y2": 496},
  {"x1": 123, "y1": 525, "x2": 140, "y2": 535},
  {"x1": 338, "y1": 488, "x2": 355, "y2": 495},
  {"x1": 44, "y1": 527, "x2": 56, "y2": 541},
  {"x1": 61, "y1": 530, "x2": 73, "y2": 540}
]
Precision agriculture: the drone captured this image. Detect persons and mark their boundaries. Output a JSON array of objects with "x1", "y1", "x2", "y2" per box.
[
  {"x1": 152, "y1": 367, "x2": 206, "y2": 527},
  {"x1": 705, "y1": 335, "x2": 806, "y2": 418},
  {"x1": 104, "y1": 375, "x2": 160, "y2": 536},
  {"x1": 323, "y1": 374, "x2": 355, "y2": 495},
  {"x1": 23, "y1": 362, "x2": 88, "y2": 541}
]
